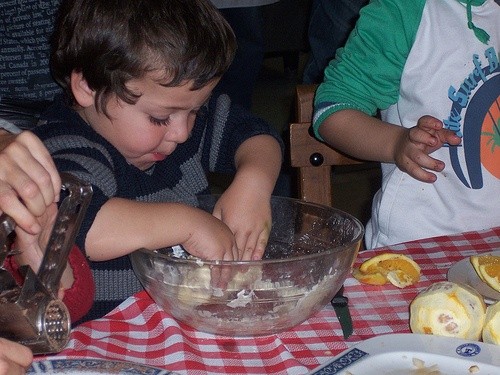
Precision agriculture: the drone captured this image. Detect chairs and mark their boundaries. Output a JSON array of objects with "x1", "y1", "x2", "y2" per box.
[{"x1": 287, "y1": 80, "x2": 382, "y2": 254}]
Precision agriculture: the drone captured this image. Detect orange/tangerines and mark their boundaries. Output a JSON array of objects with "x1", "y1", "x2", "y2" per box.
[
  {"x1": 408, "y1": 281, "x2": 500, "y2": 347},
  {"x1": 354, "y1": 256, "x2": 421, "y2": 287},
  {"x1": 471, "y1": 254, "x2": 500, "y2": 295}
]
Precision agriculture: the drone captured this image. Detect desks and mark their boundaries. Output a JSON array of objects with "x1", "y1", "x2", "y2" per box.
[{"x1": 26, "y1": 225, "x2": 500, "y2": 375}]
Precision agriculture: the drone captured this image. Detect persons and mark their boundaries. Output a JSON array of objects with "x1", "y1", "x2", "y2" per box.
[
  {"x1": 0, "y1": 128, "x2": 95, "y2": 375},
  {"x1": 313, "y1": 0, "x2": 500, "y2": 249},
  {"x1": 302, "y1": 0, "x2": 369, "y2": 84},
  {"x1": 31, "y1": 0, "x2": 284, "y2": 332}
]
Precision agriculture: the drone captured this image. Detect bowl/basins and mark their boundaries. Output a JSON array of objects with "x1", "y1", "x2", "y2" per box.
[{"x1": 129, "y1": 192, "x2": 365, "y2": 337}]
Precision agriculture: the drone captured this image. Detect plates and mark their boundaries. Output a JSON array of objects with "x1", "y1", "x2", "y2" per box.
[
  {"x1": 310, "y1": 331, "x2": 500, "y2": 375},
  {"x1": 25, "y1": 358, "x2": 180, "y2": 375},
  {"x1": 448, "y1": 251, "x2": 500, "y2": 304}
]
